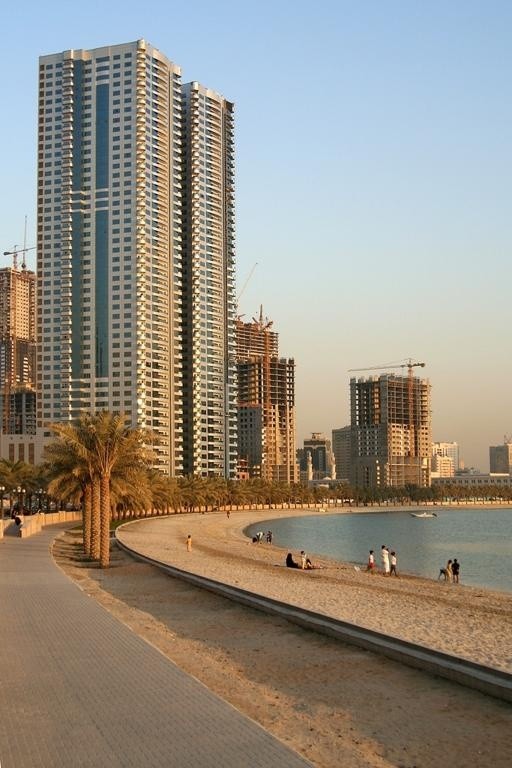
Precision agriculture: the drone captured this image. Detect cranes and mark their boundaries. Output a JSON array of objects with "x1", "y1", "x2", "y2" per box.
[
  {"x1": 2, "y1": 243, "x2": 38, "y2": 269},
  {"x1": 344, "y1": 356, "x2": 428, "y2": 484},
  {"x1": 244, "y1": 317, "x2": 275, "y2": 487}
]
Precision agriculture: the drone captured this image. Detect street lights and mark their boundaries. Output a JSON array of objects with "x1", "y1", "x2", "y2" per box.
[{"x1": 0, "y1": 484, "x2": 81, "y2": 519}]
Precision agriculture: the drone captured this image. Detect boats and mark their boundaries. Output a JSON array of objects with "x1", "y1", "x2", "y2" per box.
[{"x1": 409, "y1": 510, "x2": 441, "y2": 518}]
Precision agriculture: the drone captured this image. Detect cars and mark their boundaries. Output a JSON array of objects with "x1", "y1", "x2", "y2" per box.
[
  {"x1": 383, "y1": 498, "x2": 390, "y2": 503},
  {"x1": 284, "y1": 497, "x2": 358, "y2": 503},
  {"x1": 428, "y1": 495, "x2": 503, "y2": 501}
]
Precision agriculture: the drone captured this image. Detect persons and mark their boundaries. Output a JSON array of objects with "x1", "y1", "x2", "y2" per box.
[
  {"x1": 227, "y1": 510, "x2": 230, "y2": 519},
  {"x1": 185, "y1": 534, "x2": 193, "y2": 552},
  {"x1": 301, "y1": 550, "x2": 306, "y2": 570},
  {"x1": 378, "y1": 544, "x2": 390, "y2": 576},
  {"x1": 256, "y1": 532, "x2": 265, "y2": 544},
  {"x1": 12, "y1": 508, "x2": 42, "y2": 519},
  {"x1": 266, "y1": 530, "x2": 272, "y2": 543},
  {"x1": 451, "y1": 558, "x2": 459, "y2": 584},
  {"x1": 438, "y1": 561, "x2": 446, "y2": 580},
  {"x1": 444, "y1": 559, "x2": 452, "y2": 583},
  {"x1": 390, "y1": 551, "x2": 396, "y2": 575},
  {"x1": 364, "y1": 551, "x2": 376, "y2": 575},
  {"x1": 305, "y1": 558, "x2": 323, "y2": 569},
  {"x1": 286, "y1": 553, "x2": 298, "y2": 567}
]
